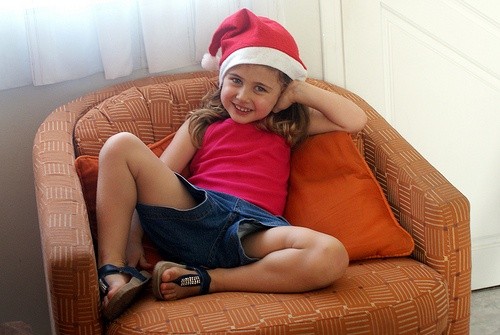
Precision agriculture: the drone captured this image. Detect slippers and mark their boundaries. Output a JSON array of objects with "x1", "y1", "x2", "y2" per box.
[
  {"x1": 154, "y1": 259, "x2": 209, "y2": 301},
  {"x1": 98, "y1": 264, "x2": 153, "y2": 321}
]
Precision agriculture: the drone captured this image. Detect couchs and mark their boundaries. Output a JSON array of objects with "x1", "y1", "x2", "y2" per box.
[{"x1": 32, "y1": 71, "x2": 472, "y2": 335}]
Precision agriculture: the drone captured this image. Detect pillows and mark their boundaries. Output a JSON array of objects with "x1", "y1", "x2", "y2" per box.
[
  {"x1": 74, "y1": 132, "x2": 191, "y2": 266},
  {"x1": 283, "y1": 130, "x2": 414, "y2": 261}
]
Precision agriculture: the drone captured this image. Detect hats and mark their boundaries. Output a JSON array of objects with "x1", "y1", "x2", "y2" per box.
[{"x1": 201, "y1": 8, "x2": 308, "y2": 89}]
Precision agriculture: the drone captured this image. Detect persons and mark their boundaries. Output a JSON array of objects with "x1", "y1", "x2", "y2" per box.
[{"x1": 96, "y1": 8, "x2": 367, "y2": 320}]
